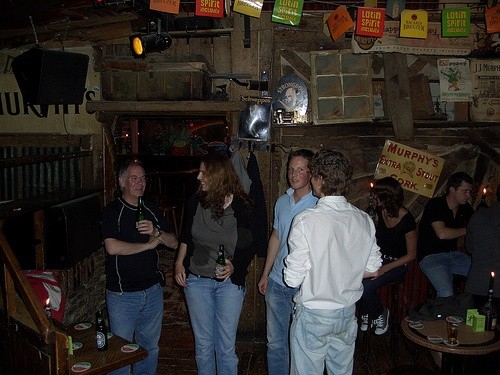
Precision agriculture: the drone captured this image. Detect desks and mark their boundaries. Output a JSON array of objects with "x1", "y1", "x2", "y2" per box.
[
  {"x1": 146, "y1": 168, "x2": 199, "y2": 238},
  {"x1": 400, "y1": 316, "x2": 500, "y2": 369},
  {"x1": 67, "y1": 321, "x2": 148, "y2": 375}
]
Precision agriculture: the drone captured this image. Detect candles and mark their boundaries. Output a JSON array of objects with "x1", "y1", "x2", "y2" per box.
[
  {"x1": 489, "y1": 272, "x2": 495, "y2": 290},
  {"x1": 369, "y1": 182, "x2": 373, "y2": 197}
]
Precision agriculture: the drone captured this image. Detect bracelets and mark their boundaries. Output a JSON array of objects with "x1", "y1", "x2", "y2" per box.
[{"x1": 154, "y1": 226, "x2": 162, "y2": 237}]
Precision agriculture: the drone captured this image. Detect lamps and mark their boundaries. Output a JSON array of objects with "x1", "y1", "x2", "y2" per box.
[{"x1": 130, "y1": 19, "x2": 173, "y2": 59}]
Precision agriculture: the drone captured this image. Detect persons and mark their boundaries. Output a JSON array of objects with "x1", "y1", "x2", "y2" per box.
[
  {"x1": 463, "y1": 184, "x2": 500, "y2": 324},
  {"x1": 258, "y1": 149, "x2": 320, "y2": 375},
  {"x1": 359, "y1": 176, "x2": 417, "y2": 335},
  {"x1": 173, "y1": 150, "x2": 260, "y2": 374},
  {"x1": 283, "y1": 151, "x2": 383, "y2": 375},
  {"x1": 102, "y1": 158, "x2": 178, "y2": 375},
  {"x1": 417, "y1": 171, "x2": 474, "y2": 296},
  {"x1": 282, "y1": 88, "x2": 298, "y2": 104}
]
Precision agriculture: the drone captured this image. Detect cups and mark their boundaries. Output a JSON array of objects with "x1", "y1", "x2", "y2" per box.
[{"x1": 447, "y1": 321, "x2": 459, "y2": 344}]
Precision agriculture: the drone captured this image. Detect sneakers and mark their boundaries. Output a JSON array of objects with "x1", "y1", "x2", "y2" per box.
[
  {"x1": 373, "y1": 306, "x2": 390, "y2": 335},
  {"x1": 361, "y1": 313, "x2": 373, "y2": 331}
]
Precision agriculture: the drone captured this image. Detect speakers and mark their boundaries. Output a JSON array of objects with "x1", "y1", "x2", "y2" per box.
[{"x1": 11, "y1": 48, "x2": 89, "y2": 105}]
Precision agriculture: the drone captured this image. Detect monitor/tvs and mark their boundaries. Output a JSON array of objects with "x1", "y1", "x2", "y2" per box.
[{"x1": 40, "y1": 190, "x2": 102, "y2": 269}]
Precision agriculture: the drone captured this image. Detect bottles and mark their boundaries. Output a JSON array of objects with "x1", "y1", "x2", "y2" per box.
[
  {"x1": 216, "y1": 244, "x2": 225, "y2": 282},
  {"x1": 97, "y1": 309, "x2": 108, "y2": 351},
  {"x1": 483, "y1": 290, "x2": 494, "y2": 330},
  {"x1": 476, "y1": 194, "x2": 486, "y2": 211},
  {"x1": 137, "y1": 196, "x2": 145, "y2": 235}
]
